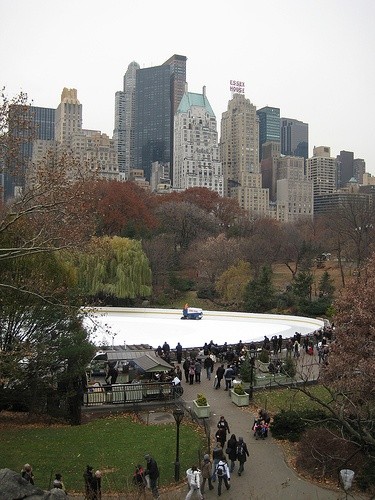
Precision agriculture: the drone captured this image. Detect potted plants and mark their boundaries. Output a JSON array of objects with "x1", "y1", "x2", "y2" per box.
[
  {"x1": 193, "y1": 393, "x2": 210, "y2": 418},
  {"x1": 258, "y1": 349, "x2": 270, "y2": 372},
  {"x1": 230, "y1": 385, "x2": 249, "y2": 407}
]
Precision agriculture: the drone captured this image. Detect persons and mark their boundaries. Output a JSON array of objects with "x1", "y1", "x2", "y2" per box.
[
  {"x1": 236, "y1": 437, "x2": 249, "y2": 476},
  {"x1": 201, "y1": 454, "x2": 214, "y2": 494},
  {"x1": 227, "y1": 434, "x2": 238, "y2": 473},
  {"x1": 83, "y1": 464, "x2": 94, "y2": 499},
  {"x1": 216, "y1": 454, "x2": 231, "y2": 496},
  {"x1": 262, "y1": 321, "x2": 334, "y2": 364},
  {"x1": 185, "y1": 465, "x2": 204, "y2": 500},
  {"x1": 132, "y1": 372, "x2": 172, "y2": 386},
  {"x1": 52, "y1": 474, "x2": 69, "y2": 494},
  {"x1": 215, "y1": 425, "x2": 226, "y2": 448},
  {"x1": 217, "y1": 416, "x2": 231, "y2": 433},
  {"x1": 157, "y1": 339, "x2": 257, "y2": 391},
  {"x1": 143, "y1": 454, "x2": 160, "y2": 500},
  {"x1": 133, "y1": 465, "x2": 150, "y2": 500},
  {"x1": 252, "y1": 410, "x2": 270, "y2": 440},
  {"x1": 213, "y1": 442, "x2": 227, "y2": 483},
  {"x1": 21, "y1": 463, "x2": 35, "y2": 485},
  {"x1": 90, "y1": 470, "x2": 102, "y2": 500}
]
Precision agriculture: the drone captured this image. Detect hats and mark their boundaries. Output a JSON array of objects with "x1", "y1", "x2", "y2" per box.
[
  {"x1": 24, "y1": 463, "x2": 32, "y2": 473},
  {"x1": 144, "y1": 454, "x2": 152, "y2": 463},
  {"x1": 95, "y1": 470, "x2": 102, "y2": 479},
  {"x1": 204, "y1": 454, "x2": 209, "y2": 460}
]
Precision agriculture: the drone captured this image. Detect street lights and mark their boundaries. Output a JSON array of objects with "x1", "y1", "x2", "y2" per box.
[{"x1": 172, "y1": 408, "x2": 185, "y2": 482}]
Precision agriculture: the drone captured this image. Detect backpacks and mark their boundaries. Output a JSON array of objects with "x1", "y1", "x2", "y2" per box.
[
  {"x1": 236, "y1": 446, "x2": 245, "y2": 456},
  {"x1": 226, "y1": 447, "x2": 233, "y2": 454},
  {"x1": 217, "y1": 469, "x2": 223, "y2": 475}
]
